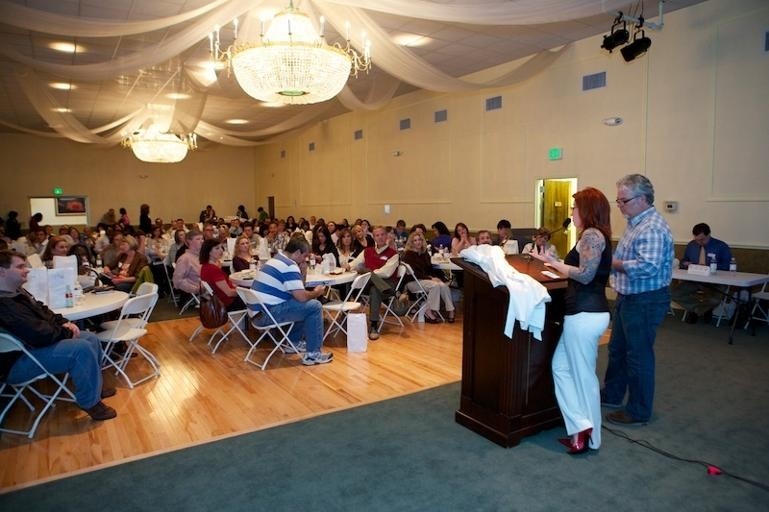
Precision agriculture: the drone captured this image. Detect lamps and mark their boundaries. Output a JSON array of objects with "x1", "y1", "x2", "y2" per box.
[
  {"x1": 208, "y1": 0, "x2": 372, "y2": 105},
  {"x1": 599, "y1": 1, "x2": 665, "y2": 64},
  {"x1": 118, "y1": 115, "x2": 198, "y2": 163}
]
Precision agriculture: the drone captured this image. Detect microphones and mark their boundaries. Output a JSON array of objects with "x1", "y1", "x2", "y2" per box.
[{"x1": 520, "y1": 218, "x2": 571, "y2": 258}]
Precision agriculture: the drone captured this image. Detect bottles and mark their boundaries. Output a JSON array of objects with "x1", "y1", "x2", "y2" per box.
[
  {"x1": 65, "y1": 254, "x2": 105, "y2": 307},
  {"x1": 709, "y1": 254, "x2": 717, "y2": 275},
  {"x1": 248, "y1": 257, "x2": 255, "y2": 271},
  {"x1": 729, "y1": 257, "x2": 736, "y2": 276},
  {"x1": 426, "y1": 243, "x2": 450, "y2": 260},
  {"x1": 308, "y1": 252, "x2": 315, "y2": 272}
]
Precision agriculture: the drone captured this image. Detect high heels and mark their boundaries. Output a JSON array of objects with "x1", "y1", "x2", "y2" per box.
[
  {"x1": 566, "y1": 428, "x2": 594, "y2": 455},
  {"x1": 558, "y1": 437, "x2": 574, "y2": 448},
  {"x1": 447, "y1": 310, "x2": 455, "y2": 323},
  {"x1": 424, "y1": 312, "x2": 442, "y2": 324}
]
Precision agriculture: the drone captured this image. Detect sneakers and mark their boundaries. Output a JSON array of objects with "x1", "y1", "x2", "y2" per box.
[
  {"x1": 284, "y1": 338, "x2": 308, "y2": 353},
  {"x1": 301, "y1": 350, "x2": 333, "y2": 366},
  {"x1": 101, "y1": 386, "x2": 116, "y2": 399},
  {"x1": 83, "y1": 400, "x2": 117, "y2": 421}
]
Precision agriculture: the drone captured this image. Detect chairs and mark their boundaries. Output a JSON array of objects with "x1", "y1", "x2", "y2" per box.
[
  {"x1": 2, "y1": 333, "x2": 83, "y2": 441},
  {"x1": 96, "y1": 293, "x2": 160, "y2": 389},
  {"x1": 2, "y1": 216, "x2": 145, "y2": 299},
  {"x1": 100, "y1": 282, "x2": 162, "y2": 376},
  {"x1": 666, "y1": 258, "x2": 681, "y2": 315},
  {"x1": 681, "y1": 283, "x2": 730, "y2": 328},
  {"x1": 146, "y1": 214, "x2": 468, "y2": 371},
  {"x1": 743, "y1": 282, "x2": 769, "y2": 331}
]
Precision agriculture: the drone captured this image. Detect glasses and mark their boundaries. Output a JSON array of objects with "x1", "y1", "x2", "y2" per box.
[
  {"x1": 569, "y1": 205, "x2": 578, "y2": 212},
  {"x1": 615, "y1": 195, "x2": 637, "y2": 206}
]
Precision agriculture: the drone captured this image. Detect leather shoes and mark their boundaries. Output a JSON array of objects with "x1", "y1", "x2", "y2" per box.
[
  {"x1": 369, "y1": 325, "x2": 379, "y2": 340},
  {"x1": 604, "y1": 409, "x2": 633, "y2": 423},
  {"x1": 599, "y1": 389, "x2": 623, "y2": 408},
  {"x1": 397, "y1": 294, "x2": 407, "y2": 304}
]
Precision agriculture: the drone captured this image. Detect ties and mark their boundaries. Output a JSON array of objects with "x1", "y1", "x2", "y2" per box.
[{"x1": 698, "y1": 246, "x2": 706, "y2": 265}]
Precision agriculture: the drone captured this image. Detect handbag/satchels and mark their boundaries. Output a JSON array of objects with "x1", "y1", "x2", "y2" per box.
[{"x1": 199, "y1": 292, "x2": 229, "y2": 329}]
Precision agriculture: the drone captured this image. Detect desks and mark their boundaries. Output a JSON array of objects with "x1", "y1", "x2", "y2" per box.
[
  {"x1": 35, "y1": 290, "x2": 130, "y2": 365},
  {"x1": 666, "y1": 263, "x2": 769, "y2": 346}
]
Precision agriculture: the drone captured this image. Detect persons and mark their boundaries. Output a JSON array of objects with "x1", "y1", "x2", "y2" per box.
[
  {"x1": 600, "y1": 173, "x2": 676, "y2": 428},
  {"x1": 528, "y1": 186, "x2": 612, "y2": 455},
  {"x1": 671, "y1": 222, "x2": 732, "y2": 324}
]
